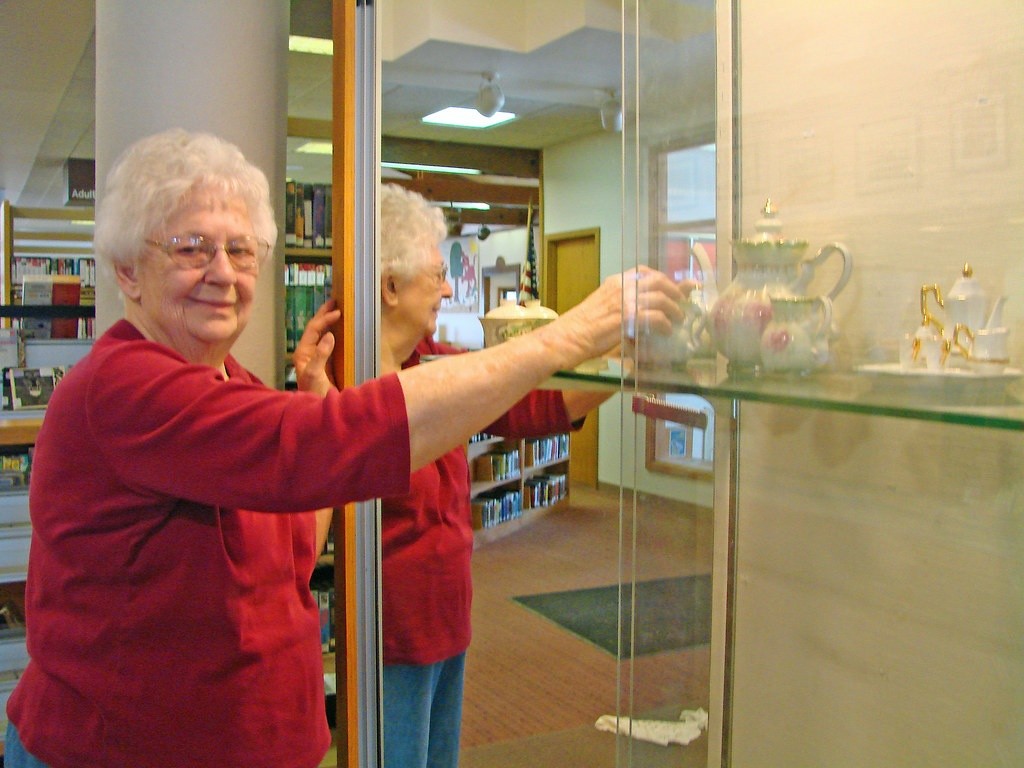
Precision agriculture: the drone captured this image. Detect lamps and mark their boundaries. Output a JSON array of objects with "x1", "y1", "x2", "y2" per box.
[
  {"x1": 477, "y1": 223, "x2": 490, "y2": 240},
  {"x1": 599, "y1": 87, "x2": 624, "y2": 132},
  {"x1": 472, "y1": 71, "x2": 506, "y2": 118}
]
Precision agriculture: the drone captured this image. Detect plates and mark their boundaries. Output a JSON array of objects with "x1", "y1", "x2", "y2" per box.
[{"x1": 854, "y1": 363, "x2": 1021, "y2": 385}]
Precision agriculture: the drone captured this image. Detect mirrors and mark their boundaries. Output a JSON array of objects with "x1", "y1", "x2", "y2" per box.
[{"x1": 377, "y1": 0, "x2": 703, "y2": 768}]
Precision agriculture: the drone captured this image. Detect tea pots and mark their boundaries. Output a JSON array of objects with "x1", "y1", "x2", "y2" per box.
[
  {"x1": 920, "y1": 262, "x2": 1011, "y2": 367},
  {"x1": 692, "y1": 198, "x2": 853, "y2": 378}
]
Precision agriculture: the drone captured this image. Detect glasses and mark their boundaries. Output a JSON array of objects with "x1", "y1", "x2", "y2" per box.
[
  {"x1": 418, "y1": 261, "x2": 448, "y2": 285},
  {"x1": 144, "y1": 232, "x2": 269, "y2": 270}
]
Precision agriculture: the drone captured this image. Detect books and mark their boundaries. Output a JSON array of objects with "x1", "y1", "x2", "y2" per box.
[
  {"x1": 0, "y1": 447, "x2": 34, "y2": 487},
  {"x1": 310, "y1": 529, "x2": 335, "y2": 653},
  {"x1": 285, "y1": 263, "x2": 332, "y2": 351},
  {"x1": 285, "y1": 180, "x2": 332, "y2": 249},
  {"x1": 525, "y1": 434, "x2": 569, "y2": 467},
  {"x1": 469, "y1": 432, "x2": 493, "y2": 442},
  {"x1": 524, "y1": 473, "x2": 566, "y2": 508},
  {"x1": 11, "y1": 257, "x2": 94, "y2": 339},
  {"x1": 477, "y1": 449, "x2": 520, "y2": 480},
  {"x1": 2, "y1": 364, "x2": 73, "y2": 411},
  {"x1": 471, "y1": 488, "x2": 522, "y2": 530}
]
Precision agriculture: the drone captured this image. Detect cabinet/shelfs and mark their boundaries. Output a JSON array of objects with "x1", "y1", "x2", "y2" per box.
[
  {"x1": 466, "y1": 423, "x2": 571, "y2": 549},
  {"x1": 0, "y1": 198, "x2": 336, "y2": 768}
]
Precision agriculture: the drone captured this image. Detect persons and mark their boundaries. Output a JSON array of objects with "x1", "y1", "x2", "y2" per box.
[
  {"x1": 5, "y1": 129, "x2": 684, "y2": 768},
  {"x1": 379, "y1": 183, "x2": 703, "y2": 768}
]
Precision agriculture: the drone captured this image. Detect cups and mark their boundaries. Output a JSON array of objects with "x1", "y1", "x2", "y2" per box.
[
  {"x1": 903, "y1": 317, "x2": 953, "y2": 368},
  {"x1": 478, "y1": 300, "x2": 537, "y2": 348},
  {"x1": 522, "y1": 300, "x2": 558, "y2": 332},
  {"x1": 627, "y1": 299, "x2": 707, "y2": 371},
  {"x1": 950, "y1": 321, "x2": 1010, "y2": 362}
]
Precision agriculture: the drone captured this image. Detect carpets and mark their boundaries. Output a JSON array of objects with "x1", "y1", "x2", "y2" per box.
[
  {"x1": 456, "y1": 698, "x2": 709, "y2": 768},
  {"x1": 506, "y1": 575, "x2": 714, "y2": 659}
]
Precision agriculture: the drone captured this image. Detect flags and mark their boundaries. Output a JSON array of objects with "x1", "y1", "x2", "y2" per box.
[{"x1": 518, "y1": 218, "x2": 538, "y2": 307}]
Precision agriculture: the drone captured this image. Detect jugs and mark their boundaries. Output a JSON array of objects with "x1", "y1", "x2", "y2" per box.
[{"x1": 759, "y1": 293, "x2": 833, "y2": 373}]
